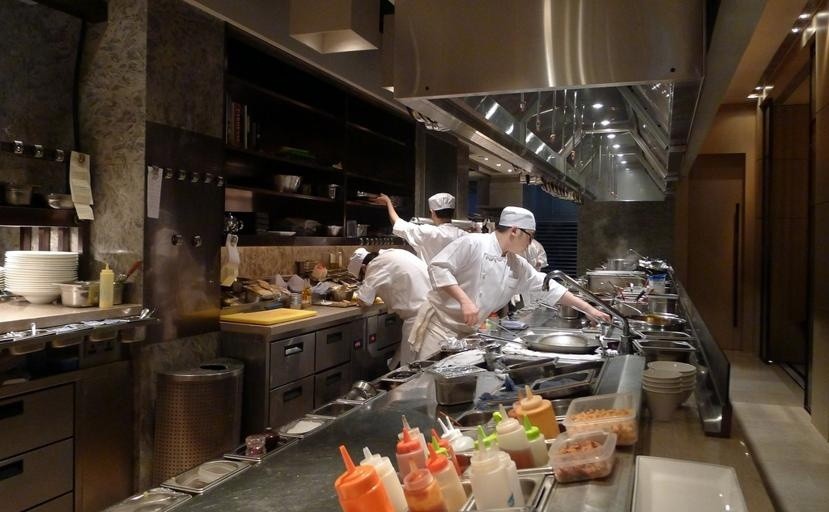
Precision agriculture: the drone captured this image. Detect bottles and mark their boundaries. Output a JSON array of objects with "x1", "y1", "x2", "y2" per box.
[
  {"x1": 224, "y1": 212, "x2": 244, "y2": 232},
  {"x1": 648, "y1": 274, "x2": 666, "y2": 296},
  {"x1": 334, "y1": 384, "x2": 558, "y2": 511},
  {"x1": 99, "y1": 264, "x2": 116, "y2": 308},
  {"x1": 489, "y1": 312, "x2": 500, "y2": 332}
]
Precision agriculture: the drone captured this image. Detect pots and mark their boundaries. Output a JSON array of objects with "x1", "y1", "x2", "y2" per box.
[
  {"x1": 646, "y1": 294, "x2": 680, "y2": 314},
  {"x1": 5, "y1": 185, "x2": 33, "y2": 204},
  {"x1": 623, "y1": 314, "x2": 688, "y2": 332},
  {"x1": 51, "y1": 279, "x2": 125, "y2": 307},
  {"x1": 607, "y1": 258, "x2": 633, "y2": 271},
  {"x1": 478, "y1": 333, "x2": 602, "y2": 353}
]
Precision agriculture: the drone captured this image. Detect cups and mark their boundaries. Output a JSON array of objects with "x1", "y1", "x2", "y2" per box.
[
  {"x1": 246, "y1": 435, "x2": 267, "y2": 456},
  {"x1": 347, "y1": 221, "x2": 357, "y2": 238},
  {"x1": 356, "y1": 224, "x2": 370, "y2": 237},
  {"x1": 290, "y1": 292, "x2": 302, "y2": 309},
  {"x1": 329, "y1": 184, "x2": 339, "y2": 199}
]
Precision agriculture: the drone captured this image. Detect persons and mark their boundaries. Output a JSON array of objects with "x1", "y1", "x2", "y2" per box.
[
  {"x1": 407, "y1": 206, "x2": 612, "y2": 360},
  {"x1": 375, "y1": 191, "x2": 469, "y2": 264},
  {"x1": 346, "y1": 247, "x2": 433, "y2": 371},
  {"x1": 508, "y1": 238, "x2": 548, "y2": 311}
]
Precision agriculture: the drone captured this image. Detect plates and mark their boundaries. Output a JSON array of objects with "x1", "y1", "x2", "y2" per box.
[
  {"x1": 267, "y1": 231, "x2": 296, "y2": 237},
  {"x1": 630, "y1": 455, "x2": 749, "y2": 512},
  {"x1": 538, "y1": 332, "x2": 589, "y2": 347},
  {"x1": 4, "y1": 250, "x2": 79, "y2": 304}
]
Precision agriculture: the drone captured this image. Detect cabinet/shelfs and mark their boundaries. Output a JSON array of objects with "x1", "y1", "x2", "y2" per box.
[
  {"x1": 225, "y1": 69, "x2": 413, "y2": 246},
  {"x1": 217, "y1": 315, "x2": 407, "y2": 446},
  {"x1": 0, "y1": 375, "x2": 75, "y2": 512}
]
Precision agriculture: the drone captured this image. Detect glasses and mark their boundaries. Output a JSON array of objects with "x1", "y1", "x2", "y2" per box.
[{"x1": 507, "y1": 227, "x2": 534, "y2": 240}]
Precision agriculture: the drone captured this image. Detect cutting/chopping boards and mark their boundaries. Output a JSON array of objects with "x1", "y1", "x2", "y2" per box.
[
  {"x1": 220, "y1": 308, "x2": 316, "y2": 325},
  {"x1": 314, "y1": 299, "x2": 360, "y2": 308}
]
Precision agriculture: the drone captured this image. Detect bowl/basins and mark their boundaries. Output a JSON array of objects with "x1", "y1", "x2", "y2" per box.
[
  {"x1": 352, "y1": 380, "x2": 377, "y2": 398},
  {"x1": 259, "y1": 427, "x2": 280, "y2": 442},
  {"x1": 642, "y1": 361, "x2": 697, "y2": 417},
  {"x1": 274, "y1": 174, "x2": 301, "y2": 192},
  {"x1": 328, "y1": 226, "x2": 342, "y2": 236},
  {"x1": 47, "y1": 194, "x2": 74, "y2": 209}
]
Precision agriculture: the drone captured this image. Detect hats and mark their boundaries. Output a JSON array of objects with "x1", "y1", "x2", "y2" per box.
[
  {"x1": 499, "y1": 206, "x2": 536, "y2": 230},
  {"x1": 348, "y1": 247, "x2": 369, "y2": 275},
  {"x1": 428, "y1": 192, "x2": 455, "y2": 211}
]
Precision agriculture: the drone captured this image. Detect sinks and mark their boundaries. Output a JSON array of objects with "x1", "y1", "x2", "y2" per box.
[{"x1": 473, "y1": 355, "x2": 593, "y2": 376}]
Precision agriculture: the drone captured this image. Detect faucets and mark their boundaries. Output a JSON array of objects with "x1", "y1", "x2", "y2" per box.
[{"x1": 542, "y1": 270, "x2": 636, "y2": 357}]
